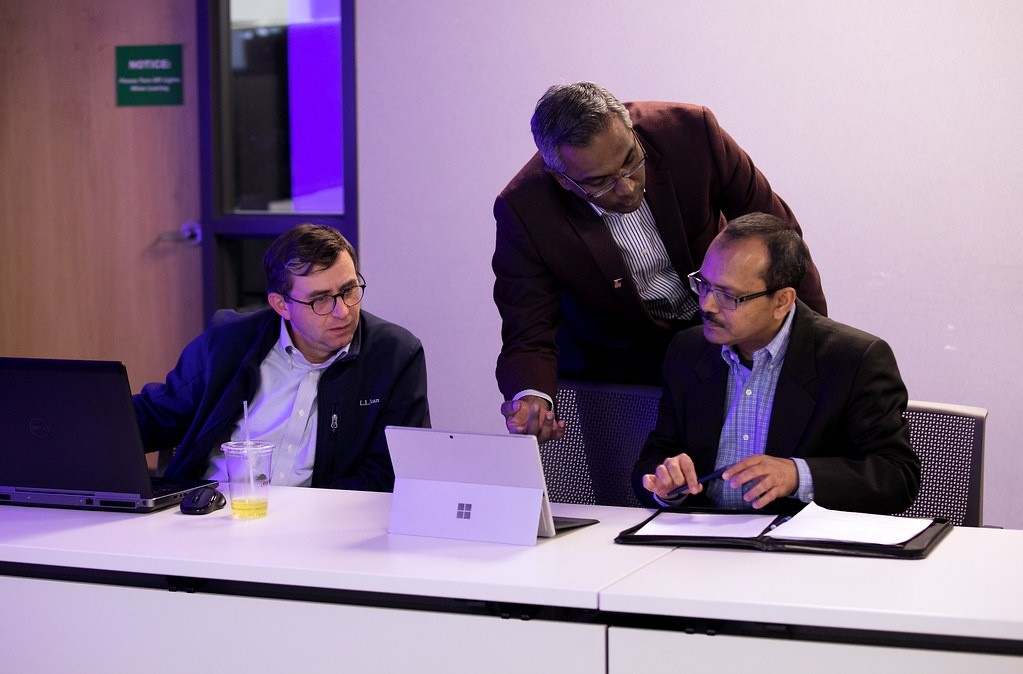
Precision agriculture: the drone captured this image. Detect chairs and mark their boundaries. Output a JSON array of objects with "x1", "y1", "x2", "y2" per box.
[
  {"x1": 521, "y1": 385, "x2": 667, "y2": 513},
  {"x1": 901, "y1": 400, "x2": 1004, "y2": 529}
]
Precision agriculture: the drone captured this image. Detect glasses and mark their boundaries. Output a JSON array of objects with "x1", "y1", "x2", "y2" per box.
[
  {"x1": 560, "y1": 128, "x2": 648, "y2": 200},
  {"x1": 687, "y1": 270, "x2": 782, "y2": 310},
  {"x1": 285, "y1": 272, "x2": 366, "y2": 315}
]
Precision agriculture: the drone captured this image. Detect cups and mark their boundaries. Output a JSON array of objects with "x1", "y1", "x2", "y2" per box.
[{"x1": 221, "y1": 440, "x2": 276, "y2": 517}]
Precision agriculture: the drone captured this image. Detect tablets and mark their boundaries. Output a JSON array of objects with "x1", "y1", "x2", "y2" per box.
[{"x1": 382, "y1": 426, "x2": 556, "y2": 539}]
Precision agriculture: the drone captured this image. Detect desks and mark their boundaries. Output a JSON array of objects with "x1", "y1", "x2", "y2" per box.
[{"x1": 1, "y1": 489, "x2": 1023, "y2": 674}]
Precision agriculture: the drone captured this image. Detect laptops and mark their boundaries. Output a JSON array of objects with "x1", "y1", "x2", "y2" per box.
[{"x1": 0, "y1": 358, "x2": 219, "y2": 512}]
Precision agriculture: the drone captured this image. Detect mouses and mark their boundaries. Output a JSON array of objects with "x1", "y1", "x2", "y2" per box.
[{"x1": 179, "y1": 488, "x2": 226, "y2": 516}]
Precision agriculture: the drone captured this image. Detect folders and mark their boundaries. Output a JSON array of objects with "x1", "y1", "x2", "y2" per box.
[{"x1": 616, "y1": 507, "x2": 955, "y2": 560}]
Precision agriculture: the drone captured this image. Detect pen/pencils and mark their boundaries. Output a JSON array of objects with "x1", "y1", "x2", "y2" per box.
[{"x1": 664, "y1": 462, "x2": 737, "y2": 499}]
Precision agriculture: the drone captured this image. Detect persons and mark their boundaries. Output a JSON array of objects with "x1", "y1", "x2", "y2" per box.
[
  {"x1": 491, "y1": 79, "x2": 829, "y2": 443},
  {"x1": 131, "y1": 223, "x2": 435, "y2": 495},
  {"x1": 632, "y1": 212, "x2": 920, "y2": 515}
]
